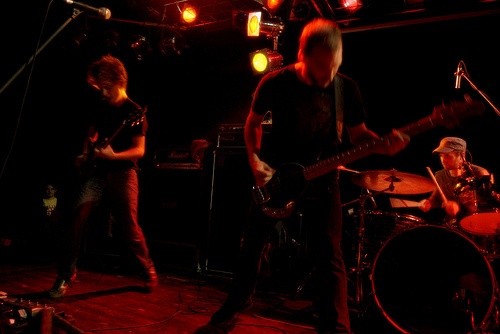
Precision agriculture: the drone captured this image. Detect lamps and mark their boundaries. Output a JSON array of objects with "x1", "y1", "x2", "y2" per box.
[{"x1": 176, "y1": 0, "x2": 364, "y2": 74}]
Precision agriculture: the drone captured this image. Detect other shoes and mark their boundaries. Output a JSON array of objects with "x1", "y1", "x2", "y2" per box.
[
  {"x1": 46, "y1": 281, "x2": 69, "y2": 298},
  {"x1": 144, "y1": 278, "x2": 161, "y2": 292}
]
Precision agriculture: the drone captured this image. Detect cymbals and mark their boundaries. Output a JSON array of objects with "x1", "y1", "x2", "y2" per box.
[
  {"x1": 351, "y1": 170, "x2": 436, "y2": 196},
  {"x1": 458, "y1": 212, "x2": 500, "y2": 237},
  {"x1": 373, "y1": 198, "x2": 420, "y2": 208}
]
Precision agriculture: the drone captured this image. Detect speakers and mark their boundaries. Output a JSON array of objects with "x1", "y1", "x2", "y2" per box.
[
  {"x1": 203, "y1": 152, "x2": 264, "y2": 277},
  {"x1": 145, "y1": 169, "x2": 209, "y2": 272}
]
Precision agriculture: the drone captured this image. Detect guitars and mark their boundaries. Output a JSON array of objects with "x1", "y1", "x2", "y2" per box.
[
  {"x1": 252, "y1": 93, "x2": 486, "y2": 220},
  {"x1": 101, "y1": 105, "x2": 146, "y2": 149}
]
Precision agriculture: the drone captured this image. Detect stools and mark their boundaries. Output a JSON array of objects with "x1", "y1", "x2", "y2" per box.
[{"x1": 197, "y1": 123, "x2": 271, "y2": 285}]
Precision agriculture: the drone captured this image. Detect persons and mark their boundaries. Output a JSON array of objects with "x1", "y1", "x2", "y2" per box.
[
  {"x1": 419, "y1": 136, "x2": 500, "y2": 231},
  {"x1": 40, "y1": 183, "x2": 66, "y2": 224},
  {"x1": 195, "y1": 17, "x2": 410, "y2": 334},
  {"x1": 45, "y1": 54, "x2": 159, "y2": 297}
]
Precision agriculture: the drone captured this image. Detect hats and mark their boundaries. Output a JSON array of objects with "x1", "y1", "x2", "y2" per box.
[{"x1": 433, "y1": 136, "x2": 466, "y2": 153}]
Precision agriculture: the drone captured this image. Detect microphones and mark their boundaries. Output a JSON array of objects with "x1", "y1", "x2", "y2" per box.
[
  {"x1": 365, "y1": 188, "x2": 378, "y2": 209},
  {"x1": 66, "y1": 0, "x2": 112, "y2": 20},
  {"x1": 454, "y1": 61, "x2": 463, "y2": 89}
]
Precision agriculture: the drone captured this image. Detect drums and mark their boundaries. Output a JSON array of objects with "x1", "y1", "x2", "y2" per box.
[
  {"x1": 365, "y1": 212, "x2": 425, "y2": 269},
  {"x1": 370, "y1": 222, "x2": 499, "y2": 334}
]
